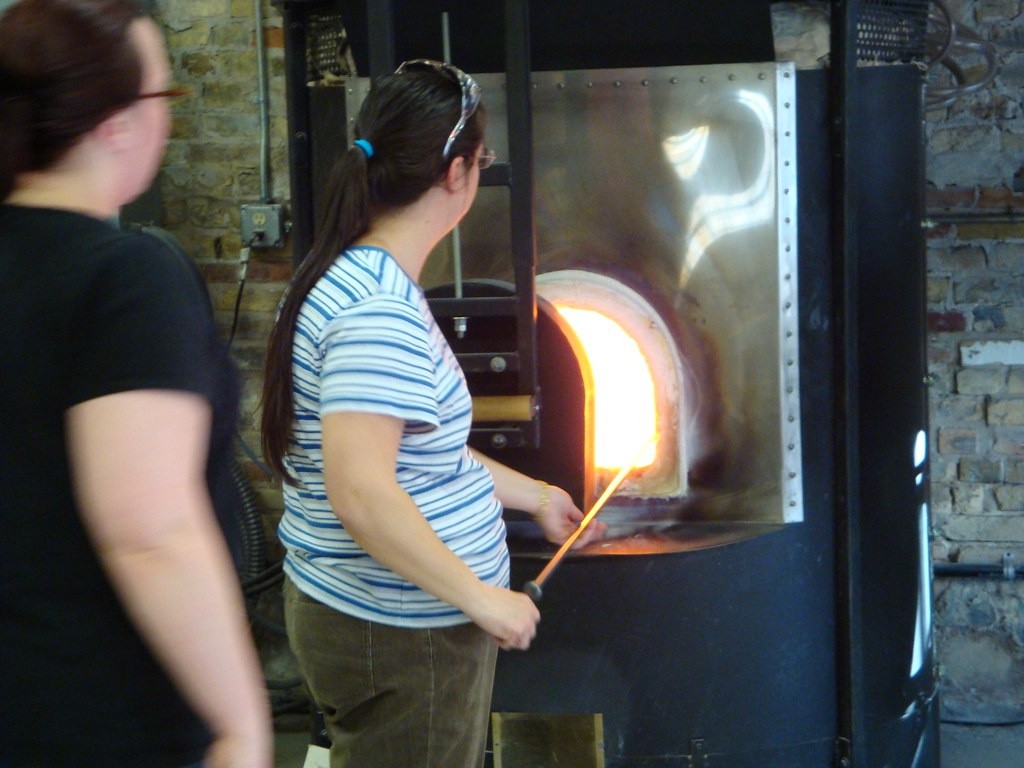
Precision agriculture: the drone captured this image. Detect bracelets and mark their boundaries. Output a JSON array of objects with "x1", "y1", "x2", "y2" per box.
[{"x1": 533, "y1": 480, "x2": 549, "y2": 525}]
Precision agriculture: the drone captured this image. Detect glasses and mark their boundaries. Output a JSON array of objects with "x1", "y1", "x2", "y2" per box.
[
  {"x1": 133, "y1": 74, "x2": 200, "y2": 140},
  {"x1": 396, "y1": 58, "x2": 481, "y2": 163},
  {"x1": 473, "y1": 146, "x2": 498, "y2": 169}
]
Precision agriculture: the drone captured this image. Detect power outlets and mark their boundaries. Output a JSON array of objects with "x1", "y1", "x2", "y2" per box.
[{"x1": 238, "y1": 201, "x2": 288, "y2": 250}]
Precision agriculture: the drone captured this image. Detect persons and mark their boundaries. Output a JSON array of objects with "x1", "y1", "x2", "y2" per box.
[
  {"x1": 0, "y1": 2, "x2": 273, "y2": 768},
  {"x1": 260, "y1": 58, "x2": 606, "y2": 767}
]
